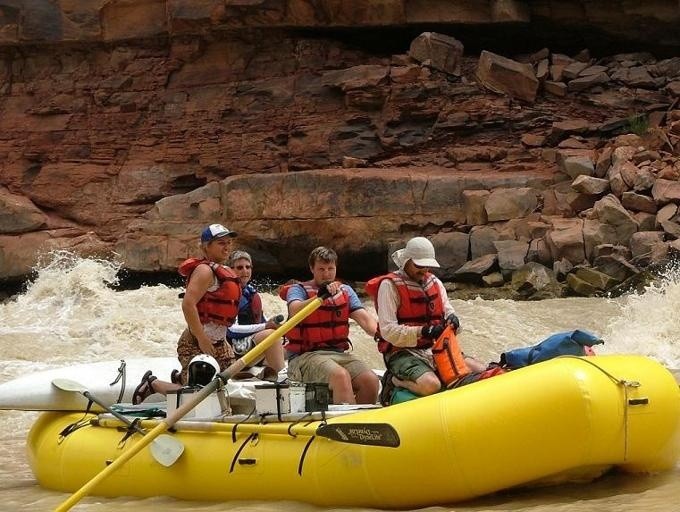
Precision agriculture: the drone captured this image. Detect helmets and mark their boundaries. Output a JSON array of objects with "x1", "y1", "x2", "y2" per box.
[{"x1": 186, "y1": 354, "x2": 221, "y2": 387}]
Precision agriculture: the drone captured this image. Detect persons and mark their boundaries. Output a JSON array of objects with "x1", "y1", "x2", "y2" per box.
[
  {"x1": 226, "y1": 250, "x2": 289, "y2": 382},
  {"x1": 280, "y1": 245, "x2": 379, "y2": 406},
  {"x1": 132, "y1": 224, "x2": 242, "y2": 407},
  {"x1": 366, "y1": 236, "x2": 486, "y2": 407}
]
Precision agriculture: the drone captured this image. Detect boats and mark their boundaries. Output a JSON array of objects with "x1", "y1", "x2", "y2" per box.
[{"x1": 25, "y1": 354, "x2": 680, "y2": 507}]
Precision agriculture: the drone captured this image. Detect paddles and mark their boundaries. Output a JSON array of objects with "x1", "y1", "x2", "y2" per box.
[
  {"x1": 98, "y1": 410, "x2": 360, "y2": 422},
  {"x1": 51, "y1": 378, "x2": 184, "y2": 468},
  {"x1": 90, "y1": 418, "x2": 400, "y2": 448}
]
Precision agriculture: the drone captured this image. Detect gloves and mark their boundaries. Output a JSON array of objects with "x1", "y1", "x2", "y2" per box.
[{"x1": 421, "y1": 324, "x2": 444, "y2": 339}]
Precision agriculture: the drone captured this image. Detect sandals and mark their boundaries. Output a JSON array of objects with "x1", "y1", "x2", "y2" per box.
[
  {"x1": 379, "y1": 369, "x2": 395, "y2": 407},
  {"x1": 132, "y1": 370, "x2": 158, "y2": 406}
]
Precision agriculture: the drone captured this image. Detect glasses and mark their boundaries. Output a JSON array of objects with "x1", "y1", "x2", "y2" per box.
[
  {"x1": 234, "y1": 265, "x2": 252, "y2": 270},
  {"x1": 413, "y1": 263, "x2": 430, "y2": 269}
]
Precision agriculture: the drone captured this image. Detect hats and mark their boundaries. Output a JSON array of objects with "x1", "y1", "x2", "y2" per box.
[
  {"x1": 405, "y1": 237, "x2": 441, "y2": 268},
  {"x1": 201, "y1": 223, "x2": 238, "y2": 243}
]
